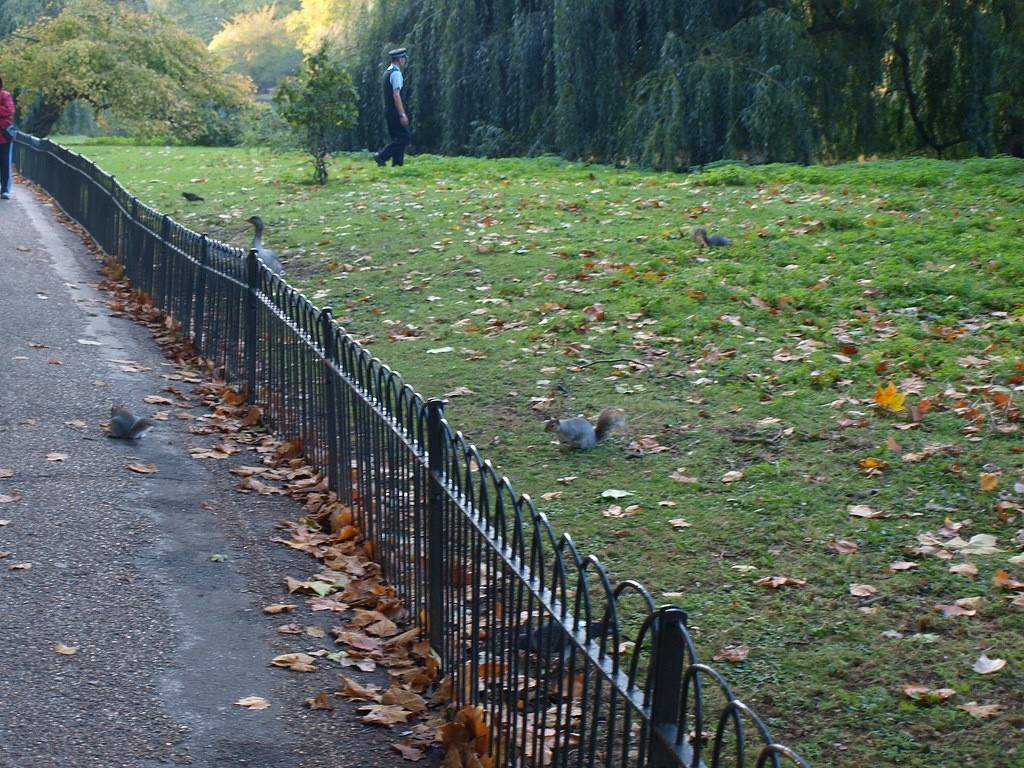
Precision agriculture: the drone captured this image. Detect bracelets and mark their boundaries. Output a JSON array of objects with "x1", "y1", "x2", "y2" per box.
[{"x1": 400, "y1": 114, "x2": 406, "y2": 118}]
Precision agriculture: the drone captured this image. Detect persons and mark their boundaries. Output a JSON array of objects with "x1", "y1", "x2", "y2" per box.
[
  {"x1": 0, "y1": 76, "x2": 15, "y2": 199},
  {"x1": 374, "y1": 48, "x2": 411, "y2": 167}
]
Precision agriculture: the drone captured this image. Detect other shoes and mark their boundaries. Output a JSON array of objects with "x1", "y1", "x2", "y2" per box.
[
  {"x1": 2, "y1": 191, "x2": 9, "y2": 200},
  {"x1": 373, "y1": 152, "x2": 386, "y2": 167}
]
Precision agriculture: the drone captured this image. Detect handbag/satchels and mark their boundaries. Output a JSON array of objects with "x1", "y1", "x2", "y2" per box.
[{"x1": 4, "y1": 124, "x2": 19, "y2": 140}]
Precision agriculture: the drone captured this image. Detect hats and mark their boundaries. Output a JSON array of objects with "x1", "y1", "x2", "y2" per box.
[{"x1": 388, "y1": 48, "x2": 409, "y2": 57}]
